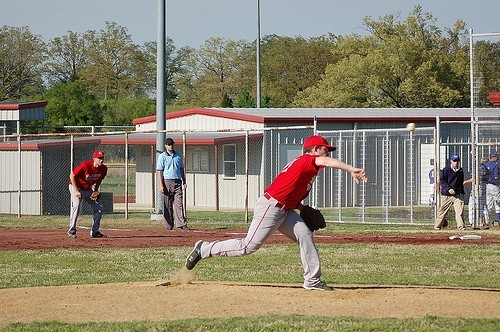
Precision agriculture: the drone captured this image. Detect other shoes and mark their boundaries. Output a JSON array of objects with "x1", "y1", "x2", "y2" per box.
[{"x1": 181, "y1": 225, "x2": 190, "y2": 230}]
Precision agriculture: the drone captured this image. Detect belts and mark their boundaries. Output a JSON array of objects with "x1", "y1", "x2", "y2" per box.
[{"x1": 265, "y1": 193, "x2": 291, "y2": 211}]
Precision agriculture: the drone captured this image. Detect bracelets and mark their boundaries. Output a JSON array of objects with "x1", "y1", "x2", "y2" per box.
[{"x1": 183, "y1": 183, "x2": 186, "y2": 185}]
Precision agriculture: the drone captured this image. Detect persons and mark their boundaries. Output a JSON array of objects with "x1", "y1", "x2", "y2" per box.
[
  {"x1": 157, "y1": 138, "x2": 191, "y2": 230},
  {"x1": 463, "y1": 157, "x2": 489, "y2": 227},
  {"x1": 68, "y1": 151, "x2": 108, "y2": 239},
  {"x1": 435, "y1": 154, "x2": 466, "y2": 231},
  {"x1": 480, "y1": 151, "x2": 500, "y2": 227},
  {"x1": 186, "y1": 136, "x2": 368, "y2": 291}
]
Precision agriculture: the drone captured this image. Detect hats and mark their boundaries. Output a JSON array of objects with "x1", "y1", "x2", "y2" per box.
[
  {"x1": 303, "y1": 135, "x2": 336, "y2": 152},
  {"x1": 491, "y1": 151, "x2": 499, "y2": 156},
  {"x1": 450, "y1": 154, "x2": 460, "y2": 161},
  {"x1": 93, "y1": 150, "x2": 107, "y2": 158}
]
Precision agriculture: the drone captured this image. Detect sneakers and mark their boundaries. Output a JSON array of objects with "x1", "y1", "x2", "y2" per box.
[
  {"x1": 304, "y1": 280, "x2": 334, "y2": 291},
  {"x1": 186, "y1": 240, "x2": 204, "y2": 270},
  {"x1": 68, "y1": 229, "x2": 76, "y2": 239},
  {"x1": 90, "y1": 231, "x2": 107, "y2": 238}
]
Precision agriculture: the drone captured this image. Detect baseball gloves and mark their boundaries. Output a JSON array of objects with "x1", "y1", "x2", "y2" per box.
[
  {"x1": 299, "y1": 205, "x2": 327, "y2": 233},
  {"x1": 90, "y1": 192, "x2": 102, "y2": 201}
]
